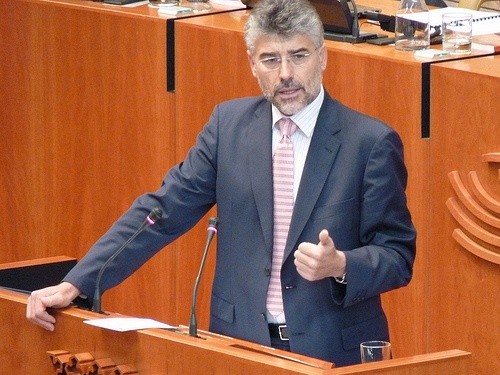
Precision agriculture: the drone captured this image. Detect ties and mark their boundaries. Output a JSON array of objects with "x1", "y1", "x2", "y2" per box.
[{"x1": 265, "y1": 119, "x2": 300, "y2": 320}]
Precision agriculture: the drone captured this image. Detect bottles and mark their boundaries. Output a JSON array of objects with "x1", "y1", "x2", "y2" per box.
[{"x1": 395, "y1": 0, "x2": 431, "y2": 51}]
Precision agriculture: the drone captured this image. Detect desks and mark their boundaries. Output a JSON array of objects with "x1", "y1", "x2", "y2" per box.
[{"x1": 1, "y1": 1, "x2": 500, "y2": 375}]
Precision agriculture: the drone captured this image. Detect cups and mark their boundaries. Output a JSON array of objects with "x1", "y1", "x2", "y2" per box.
[
  {"x1": 359, "y1": 340, "x2": 392, "y2": 364},
  {"x1": 442, "y1": 12, "x2": 473, "y2": 56},
  {"x1": 147, "y1": 0, "x2": 215, "y2": 14}
]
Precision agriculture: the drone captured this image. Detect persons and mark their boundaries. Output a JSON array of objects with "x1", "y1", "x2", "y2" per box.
[{"x1": 26, "y1": 0, "x2": 417, "y2": 367}]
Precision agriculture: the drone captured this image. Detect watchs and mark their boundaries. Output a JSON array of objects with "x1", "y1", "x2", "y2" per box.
[{"x1": 335, "y1": 271, "x2": 347, "y2": 284}]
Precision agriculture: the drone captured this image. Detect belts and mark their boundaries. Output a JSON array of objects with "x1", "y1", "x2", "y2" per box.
[{"x1": 267, "y1": 322, "x2": 290, "y2": 341}]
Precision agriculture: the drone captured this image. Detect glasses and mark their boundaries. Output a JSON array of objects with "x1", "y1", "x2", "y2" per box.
[{"x1": 251, "y1": 42, "x2": 324, "y2": 72}]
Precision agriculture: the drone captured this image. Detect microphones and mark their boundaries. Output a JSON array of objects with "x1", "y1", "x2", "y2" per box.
[
  {"x1": 189, "y1": 216, "x2": 220, "y2": 337},
  {"x1": 92, "y1": 207, "x2": 162, "y2": 312}
]
